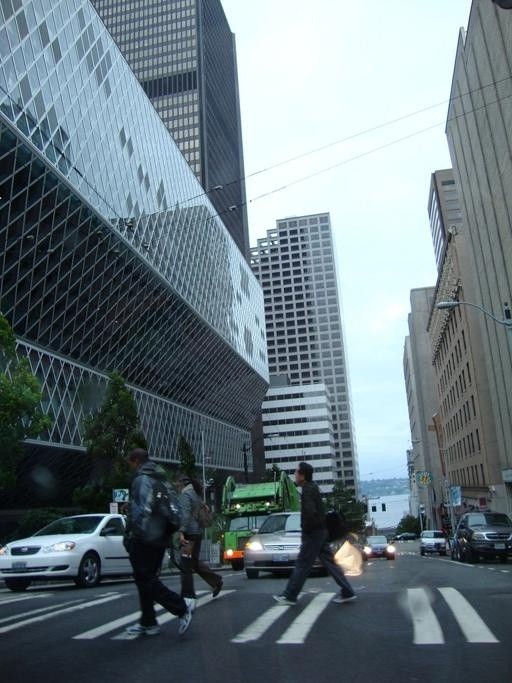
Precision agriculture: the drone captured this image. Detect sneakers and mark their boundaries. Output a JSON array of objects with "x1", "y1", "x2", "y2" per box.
[
  {"x1": 272, "y1": 594, "x2": 296, "y2": 606},
  {"x1": 126, "y1": 623, "x2": 160, "y2": 635},
  {"x1": 176, "y1": 597, "x2": 196, "y2": 636},
  {"x1": 212, "y1": 580, "x2": 223, "y2": 598},
  {"x1": 332, "y1": 594, "x2": 356, "y2": 603}
]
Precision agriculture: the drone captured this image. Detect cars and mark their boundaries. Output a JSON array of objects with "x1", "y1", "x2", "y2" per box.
[
  {"x1": 0, "y1": 513, "x2": 170, "y2": 589},
  {"x1": 244, "y1": 511, "x2": 327, "y2": 579}
]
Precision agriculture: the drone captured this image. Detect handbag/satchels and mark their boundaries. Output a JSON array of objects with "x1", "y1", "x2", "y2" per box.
[
  {"x1": 327, "y1": 512, "x2": 344, "y2": 543},
  {"x1": 188, "y1": 494, "x2": 213, "y2": 529}
]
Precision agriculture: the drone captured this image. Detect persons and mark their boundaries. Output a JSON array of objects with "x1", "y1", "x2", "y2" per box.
[
  {"x1": 122, "y1": 447, "x2": 196, "y2": 635},
  {"x1": 271, "y1": 462, "x2": 358, "y2": 606},
  {"x1": 176, "y1": 476, "x2": 225, "y2": 613}
]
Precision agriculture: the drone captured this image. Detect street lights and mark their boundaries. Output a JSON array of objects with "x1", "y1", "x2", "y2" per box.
[
  {"x1": 436, "y1": 299, "x2": 512, "y2": 336},
  {"x1": 242, "y1": 433, "x2": 280, "y2": 484}
]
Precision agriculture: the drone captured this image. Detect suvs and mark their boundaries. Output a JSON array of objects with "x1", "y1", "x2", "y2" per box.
[
  {"x1": 395, "y1": 532, "x2": 417, "y2": 541},
  {"x1": 452, "y1": 511, "x2": 512, "y2": 562},
  {"x1": 365, "y1": 535, "x2": 395, "y2": 561},
  {"x1": 420, "y1": 530, "x2": 447, "y2": 556}
]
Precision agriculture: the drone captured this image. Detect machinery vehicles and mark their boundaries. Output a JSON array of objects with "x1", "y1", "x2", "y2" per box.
[{"x1": 220, "y1": 471, "x2": 297, "y2": 571}]
